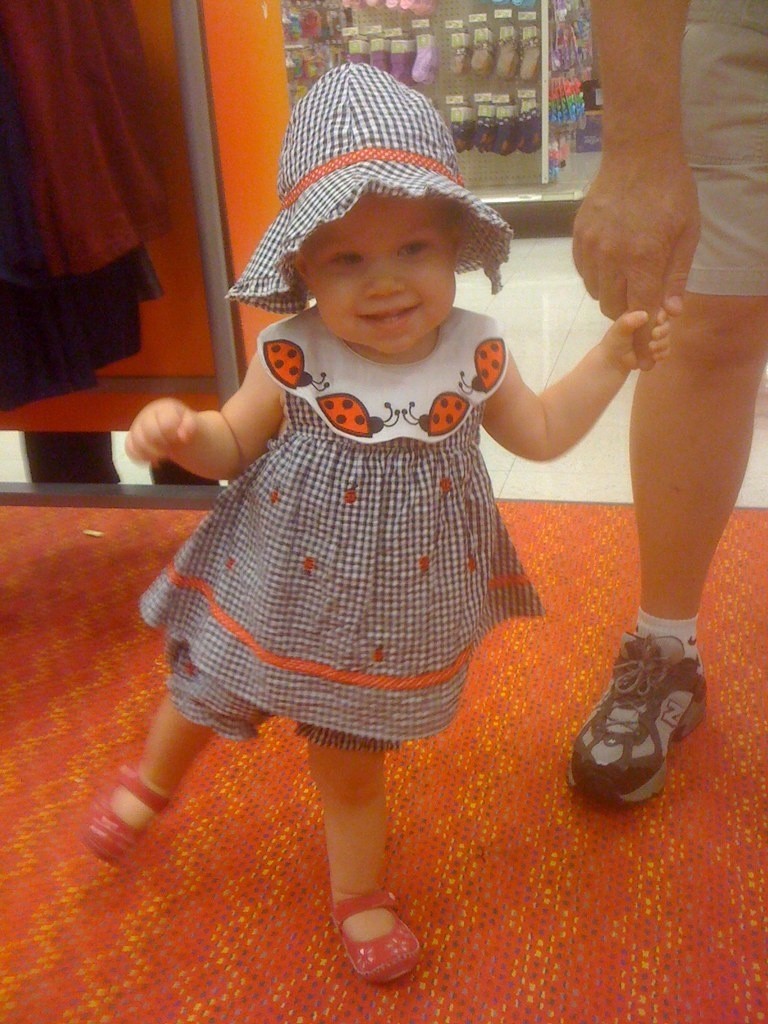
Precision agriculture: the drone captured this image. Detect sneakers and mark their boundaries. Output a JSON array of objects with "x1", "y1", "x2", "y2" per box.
[{"x1": 563, "y1": 624, "x2": 706, "y2": 802}]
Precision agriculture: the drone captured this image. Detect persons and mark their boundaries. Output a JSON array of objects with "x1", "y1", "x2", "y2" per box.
[
  {"x1": 566, "y1": 1, "x2": 768, "y2": 809},
  {"x1": 81, "y1": 63, "x2": 670, "y2": 982}
]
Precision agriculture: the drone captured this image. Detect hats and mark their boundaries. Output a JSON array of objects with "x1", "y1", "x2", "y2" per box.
[{"x1": 225, "y1": 61, "x2": 513, "y2": 315}]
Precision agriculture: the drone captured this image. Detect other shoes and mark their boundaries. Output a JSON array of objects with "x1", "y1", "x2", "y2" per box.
[
  {"x1": 77, "y1": 764, "x2": 173, "y2": 870},
  {"x1": 330, "y1": 891, "x2": 422, "y2": 983}
]
